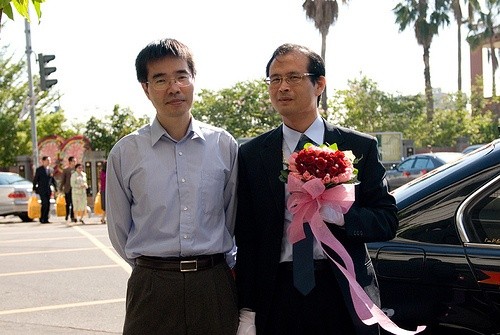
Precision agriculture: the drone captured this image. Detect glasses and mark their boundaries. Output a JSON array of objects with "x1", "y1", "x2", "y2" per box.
[
  {"x1": 264, "y1": 72, "x2": 318, "y2": 86},
  {"x1": 146, "y1": 73, "x2": 192, "y2": 91}
]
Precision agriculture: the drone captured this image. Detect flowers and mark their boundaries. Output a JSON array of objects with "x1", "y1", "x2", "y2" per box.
[{"x1": 290, "y1": 144, "x2": 360, "y2": 189}]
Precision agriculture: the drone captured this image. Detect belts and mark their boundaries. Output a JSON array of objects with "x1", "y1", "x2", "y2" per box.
[
  {"x1": 135, "y1": 253, "x2": 225, "y2": 272},
  {"x1": 280, "y1": 260, "x2": 330, "y2": 274}
]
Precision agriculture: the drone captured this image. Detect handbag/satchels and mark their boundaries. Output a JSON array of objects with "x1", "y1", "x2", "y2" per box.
[
  {"x1": 56, "y1": 194, "x2": 66, "y2": 216},
  {"x1": 28, "y1": 193, "x2": 41, "y2": 218},
  {"x1": 94, "y1": 192, "x2": 103, "y2": 215}
]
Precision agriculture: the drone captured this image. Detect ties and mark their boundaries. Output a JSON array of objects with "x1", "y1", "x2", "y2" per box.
[{"x1": 292, "y1": 135, "x2": 314, "y2": 295}]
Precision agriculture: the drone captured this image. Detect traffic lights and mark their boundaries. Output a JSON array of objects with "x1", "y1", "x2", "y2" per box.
[{"x1": 36, "y1": 53, "x2": 58, "y2": 91}]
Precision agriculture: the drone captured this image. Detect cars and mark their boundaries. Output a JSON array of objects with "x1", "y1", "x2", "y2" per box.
[
  {"x1": 231, "y1": 137, "x2": 500, "y2": 334},
  {"x1": 0, "y1": 172, "x2": 56, "y2": 222},
  {"x1": 382, "y1": 152, "x2": 466, "y2": 195}
]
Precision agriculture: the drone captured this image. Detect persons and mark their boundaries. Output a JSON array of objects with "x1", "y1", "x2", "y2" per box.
[
  {"x1": 100, "y1": 160, "x2": 107, "y2": 224},
  {"x1": 104, "y1": 38, "x2": 240, "y2": 334},
  {"x1": 32, "y1": 155, "x2": 57, "y2": 224},
  {"x1": 58, "y1": 156, "x2": 76, "y2": 220},
  {"x1": 70, "y1": 164, "x2": 89, "y2": 225},
  {"x1": 236, "y1": 44, "x2": 398, "y2": 335}
]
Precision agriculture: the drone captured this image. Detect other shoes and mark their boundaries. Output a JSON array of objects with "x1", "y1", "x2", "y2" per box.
[
  {"x1": 79, "y1": 219, "x2": 84, "y2": 225},
  {"x1": 65, "y1": 216, "x2": 68, "y2": 220},
  {"x1": 71, "y1": 218, "x2": 77, "y2": 223},
  {"x1": 101, "y1": 220, "x2": 106, "y2": 224}
]
